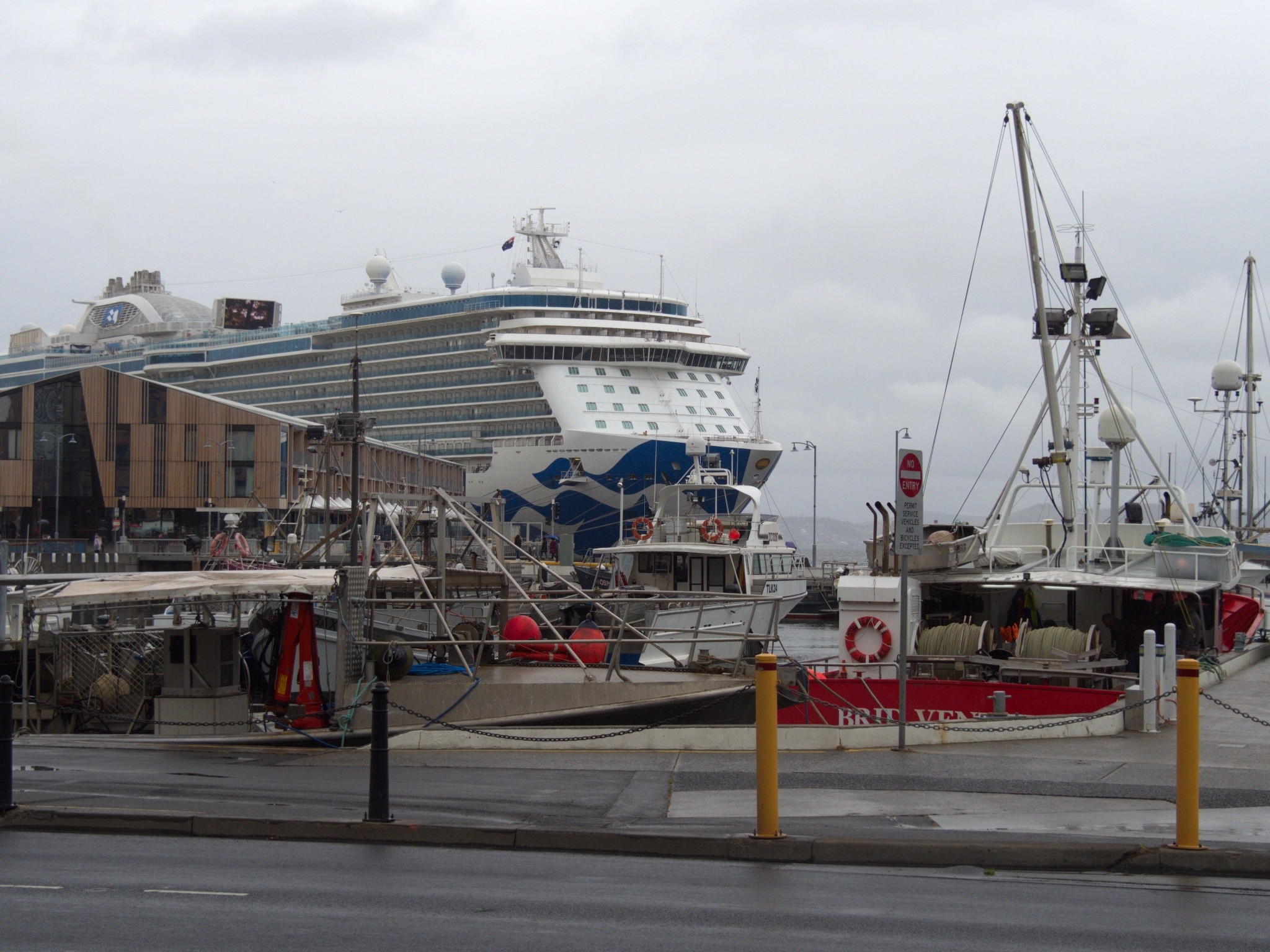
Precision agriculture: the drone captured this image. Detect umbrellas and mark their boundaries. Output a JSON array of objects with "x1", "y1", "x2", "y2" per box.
[
  {"x1": 37, "y1": 520, "x2": 50, "y2": 524},
  {"x1": 547, "y1": 535, "x2": 559, "y2": 541}
]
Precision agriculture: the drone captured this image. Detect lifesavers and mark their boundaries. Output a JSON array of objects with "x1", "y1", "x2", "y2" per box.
[
  {"x1": 631, "y1": 517, "x2": 654, "y2": 541},
  {"x1": 505, "y1": 650, "x2": 569, "y2": 663},
  {"x1": 701, "y1": 518, "x2": 724, "y2": 543},
  {"x1": 507, "y1": 639, "x2": 570, "y2": 652},
  {"x1": 210, "y1": 532, "x2": 228, "y2": 558},
  {"x1": 234, "y1": 531, "x2": 250, "y2": 557},
  {"x1": 843, "y1": 616, "x2": 892, "y2": 663}
]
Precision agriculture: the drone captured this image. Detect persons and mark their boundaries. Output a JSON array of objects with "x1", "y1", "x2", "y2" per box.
[
  {"x1": 163, "y1": 531, "x2": 171, "y2": 555},
  {"x1": 384, "y1": 533, "x2": 392, "y2": 553},
  {"x1": 41, "y1": 532, "x2": 51, "y2": 540},
  {"x1": 157, "y1": 532, "x2": 165, "y2": 556},
  {"x1": 550, "y1": 537, "x2": 558, "y2": 560},
  {"x1": 94, "y1": 533, "x2": 102, "y2": 552},
  {"x1": 1136, "y1": 592, "x2": 1178, "y2": 644},
  {"x1": 181, "y1": 523, "x2": 188, "y2": 539},
  {"x1": 1176, "y1": 598, "x2": 1202, "y2": 659},
  {"x1": 1101, "y1": 612, "x2": 1143, "y2": 672},
  {"x1": 515, "y1": 535, "x2": 521, "y2": 561},
  {"x1": 540, "y1": 537, "x2": 548, "y2": 560},
  {"x1": 41, "y1": 522, "x2": 45, "y2": 538}
]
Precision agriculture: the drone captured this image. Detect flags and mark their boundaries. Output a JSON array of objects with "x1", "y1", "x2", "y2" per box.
[{"x1": 502, "y1": 236, "x2": 515, "y2": 252}]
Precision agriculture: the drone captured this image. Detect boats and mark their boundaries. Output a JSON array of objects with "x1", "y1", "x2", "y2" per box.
[{"x1": 0, "y1": 101, "x2": 1268, "y2": 743}]
